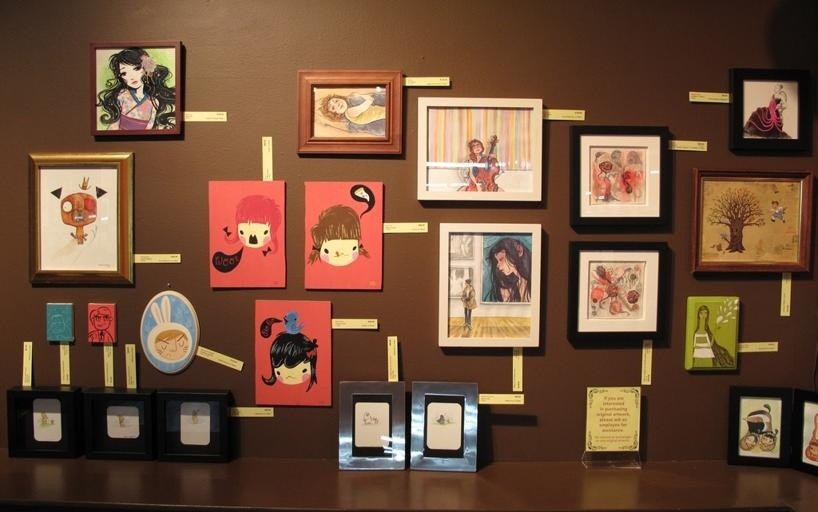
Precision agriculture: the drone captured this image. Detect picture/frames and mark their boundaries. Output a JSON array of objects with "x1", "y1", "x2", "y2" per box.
[
  {"x1": 728, "y1": 386, "x2": 818, "y2": 468},
  {"x1": 88, "y1": 40, "x2": 182, "y2": 138},
  {"x1": 28, "y1": 152, "x2": 135, "y2": 286},
  {"x1": 438, "y1": 223, "x2": 541, "y2": 349},
  {"x1": 729, "y1": 68, "x2": 812, "y2": 154},
  {"x1": 570, "y1": 240, "x2": 669, "y2": 346},
  {"x1": 338, "y1": 380, "x2": 478, "y2": 472},
  {"x1": 6, "y1": 387, "x2": 231, "y2": 458},
  {"x1": 297, "y1": 69, "x2": 404, "y2": 155},
  {"x1": 570, "y1": 125, "x2": 673, "y2": 232},
  {"x1": 417, "y1": 96, "x2": 543, "y2": 202},
  {"x1": 689, "y1": 166, "x2": 815, "y2": 275}
]
